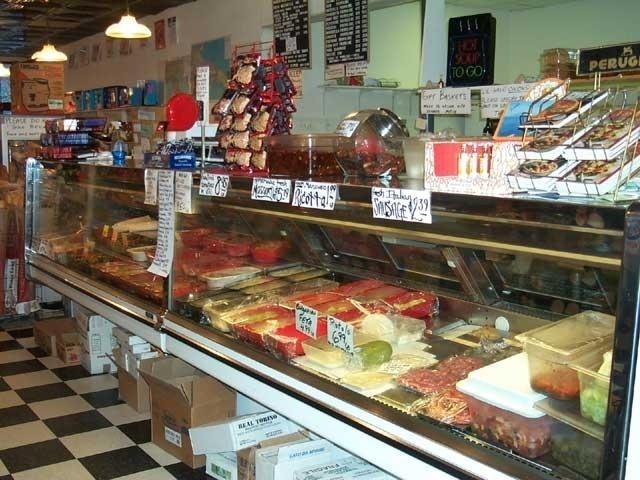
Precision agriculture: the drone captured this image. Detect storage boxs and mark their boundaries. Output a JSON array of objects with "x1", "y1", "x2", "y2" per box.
[
  {"x1": 10, "y1": 62, "x2": 66, "y2": 115},
  {"x1": 33, "y1": 303, "x2": 397, "y2": 480}
]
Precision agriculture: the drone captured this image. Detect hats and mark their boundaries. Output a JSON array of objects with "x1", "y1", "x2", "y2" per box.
[{"x1": 162, "y1": 93, "x2": 201, "y2": 132}]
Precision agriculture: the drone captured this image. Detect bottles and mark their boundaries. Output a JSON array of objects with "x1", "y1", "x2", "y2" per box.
[
  {"x1": 332, "y1": 108, "x2": 408, "y2": 179},
  {"x1": 111, "y1": 136, "x2": 127, "y2": 166}
]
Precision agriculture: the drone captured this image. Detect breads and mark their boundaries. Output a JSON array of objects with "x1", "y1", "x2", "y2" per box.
[{"x1": 541, "y1": 49, "x2": 588, "y2": 80}]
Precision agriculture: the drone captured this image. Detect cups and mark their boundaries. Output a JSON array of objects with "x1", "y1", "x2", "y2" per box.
[{"x1": 402, "y1": 139, "x2": 425, "y2": 179}]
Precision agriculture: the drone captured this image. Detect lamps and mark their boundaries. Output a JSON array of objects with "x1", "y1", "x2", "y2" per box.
[
  {"x1": 105, "y1": 1, "x2": 151, "y2": 40},
  {"x1": 29, "y1": 1, "x2": 67, "y2": 63}
]
